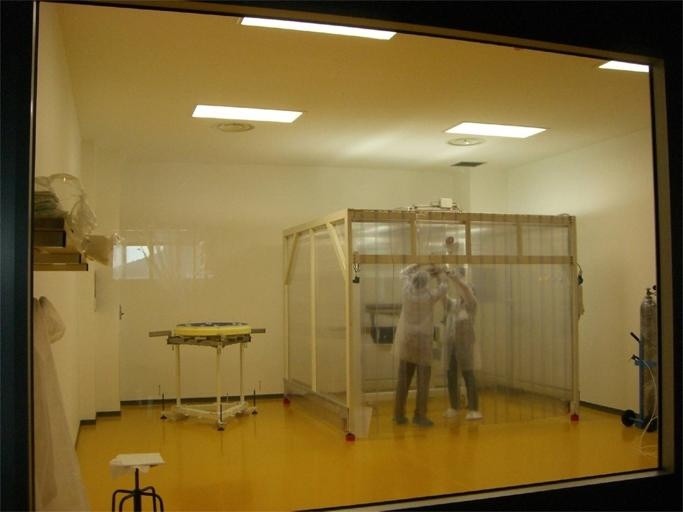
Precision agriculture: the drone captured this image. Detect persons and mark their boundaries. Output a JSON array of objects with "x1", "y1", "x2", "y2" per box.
[
  {"x1": 389, "y1": 263, "x2": 449, "y2": 428},
  {"x1": 426, "y1": 263, "x2": 483, "y2": 422}
]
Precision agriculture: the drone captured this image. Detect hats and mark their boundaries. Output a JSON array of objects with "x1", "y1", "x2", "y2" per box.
[{"x1": 412, "y1": 271, "x2": 430, "y2": 288}]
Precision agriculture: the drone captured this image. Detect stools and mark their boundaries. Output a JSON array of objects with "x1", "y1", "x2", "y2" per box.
[{"x1": 109, "y1": 453, "x2": 164, "y2": 512}]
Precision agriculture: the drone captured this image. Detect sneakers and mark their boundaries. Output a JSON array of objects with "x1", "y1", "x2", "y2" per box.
[
  {"x1": 393, "y1": 414, "x2": 408, "y2": 424},
  {"x1": 413, "y1": 416, "x2": 433, "y2": 426},
  {"x1": 465, "y1": 410, "x2": 482, "y2": 420},
  {"x1": 443, "y1": 408, "x2": 458, "y2": 417}
]
